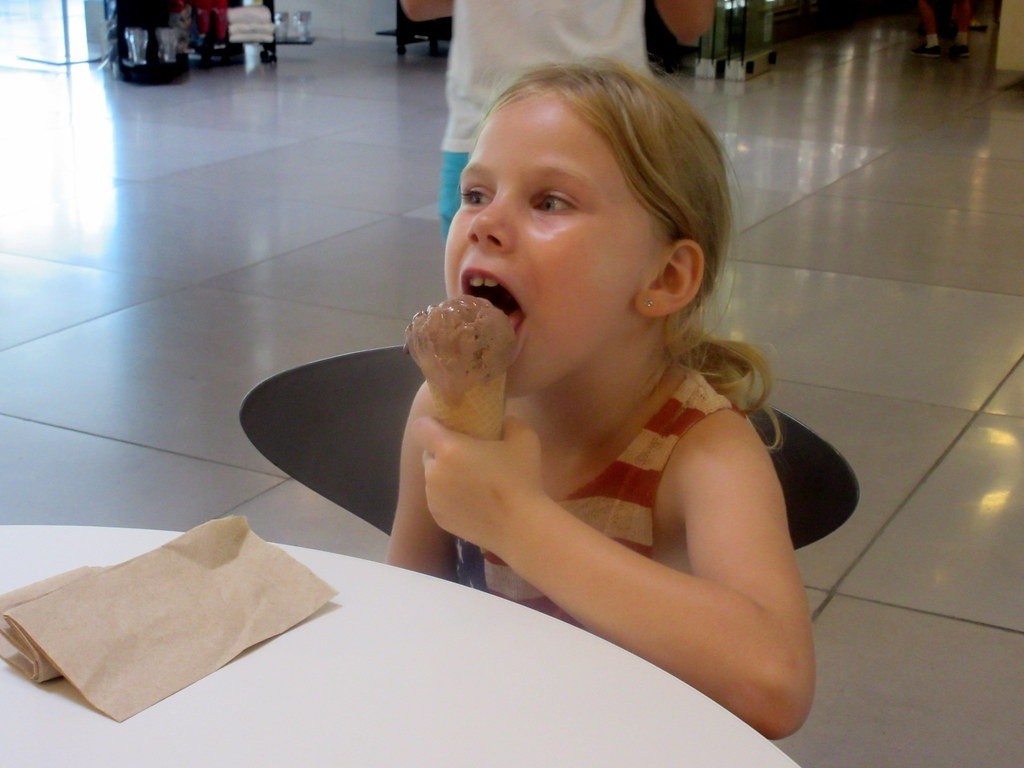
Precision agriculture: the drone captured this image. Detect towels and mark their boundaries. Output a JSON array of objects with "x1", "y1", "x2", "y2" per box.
[{"x1": 225, "y1": 4, "x2": 279, "y2": 43}]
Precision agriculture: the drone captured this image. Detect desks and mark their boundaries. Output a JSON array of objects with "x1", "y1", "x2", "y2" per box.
[{"x1": 0, "y1": 525, "x2": 802, "y2": 768}]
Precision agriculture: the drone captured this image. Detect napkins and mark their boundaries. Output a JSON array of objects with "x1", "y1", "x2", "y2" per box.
[{"x1": 0, "y1": 516, "x2": 336, "y2": 725}]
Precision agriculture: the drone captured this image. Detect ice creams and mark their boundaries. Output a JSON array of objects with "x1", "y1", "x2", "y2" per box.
[{"x1": 402, "y1": 294, "x2": 516, "y2": 441}]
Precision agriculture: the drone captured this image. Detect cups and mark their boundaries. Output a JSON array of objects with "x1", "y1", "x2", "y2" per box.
[
  {"x1": 124, "y1": 27, "x2": 140, "y2": 59},
  {"x1": 156, "y1": 29, "x2": 168, "y2": 58},
  {"x1": 274, "y1": 12, "x2": 289, "y2": 42},
  {"x1": 294, "y1": 11, "x2": 312, "y2": 41},
  {"x1": 130, "y1": 30, "x2": 148, "y2": 64},
  {"x1": 161, "y1": 30, "x2": 179, "y2": 62}
]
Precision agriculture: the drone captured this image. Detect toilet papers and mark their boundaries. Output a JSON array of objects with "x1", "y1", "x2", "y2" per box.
[{"x1": 0, "y1": 513, "x2": 338, "y2": 721}]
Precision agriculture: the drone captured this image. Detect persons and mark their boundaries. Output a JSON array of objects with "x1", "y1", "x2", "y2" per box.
[
  {"x1": 399, "y1": 0, "x2": 715, "y2": 244},
  {"x1": 909, "y1": 0, "x2": 973, "y2": 57},
  {"x1": 386, "y1": 58, "x2": 815, "y2": 741}
]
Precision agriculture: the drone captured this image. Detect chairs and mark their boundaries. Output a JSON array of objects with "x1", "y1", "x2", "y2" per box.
[{"x1": 239, "y1": 349, "x2": 858, "y2": 592}]
